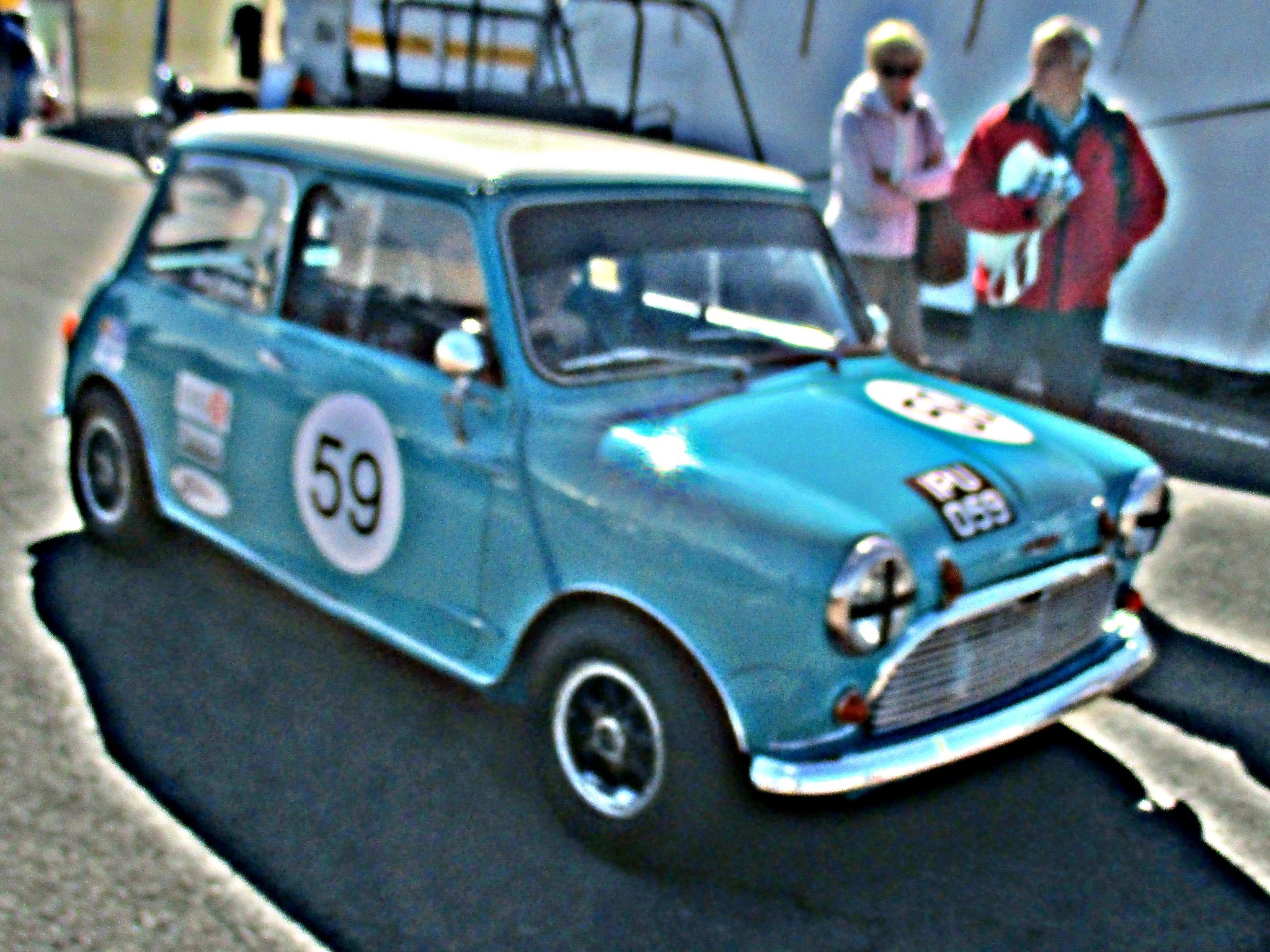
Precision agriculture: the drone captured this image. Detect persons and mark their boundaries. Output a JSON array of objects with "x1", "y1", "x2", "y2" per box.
[
  {"x1": 820, "y1": 19, "x2": 954, "y2": 369},
  {"x1": 0, "y1": 0, "x2": 62, "y2": 140},
  {"x1": 233, "y1": 0, "x2": 352, "y2": 113},
  {"x1": 949, "y1": 12, "x2": 1167, "y2": 423}
]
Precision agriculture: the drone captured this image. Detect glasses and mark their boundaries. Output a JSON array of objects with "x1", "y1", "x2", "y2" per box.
[{"x1": 880, "y1": 63, "x2": 918, "y2": 78}]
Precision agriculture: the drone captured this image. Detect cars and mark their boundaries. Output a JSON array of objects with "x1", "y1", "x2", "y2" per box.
[{"x1": 58, "y1": 112, "x2": 1174, "y2": 861}]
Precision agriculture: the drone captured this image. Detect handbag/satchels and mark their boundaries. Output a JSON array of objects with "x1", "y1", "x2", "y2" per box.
[
  {"x1": 970, "y1": 139, "x2": 1086, "y2": 308},
  {"x1": 920, "y1": 153, "x2": 966, "y2": 285}
]
